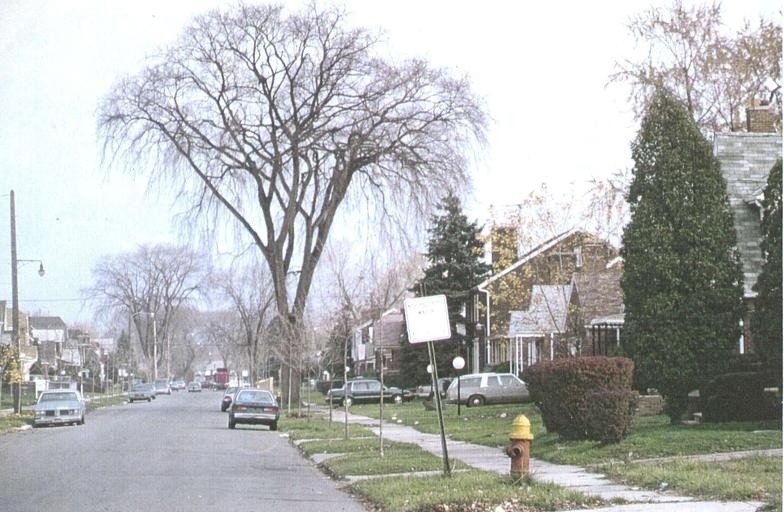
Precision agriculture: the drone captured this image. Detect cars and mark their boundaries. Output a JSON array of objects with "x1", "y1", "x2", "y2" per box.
[
  {"x1": 31, "y1": 388, "x2": 85, "y2": 428},
  {"x1": 220, "y1": 384, "x2": 281, "y2": 431},
  {"x1": 325, "y1": 378, "x2": 411, "y2": 407},
  {"x1": 413, "y1": 372, "x2": 533, "y2": 404},
  {"x1": 128, "y1": 379, "x2": 186, "y2": 403},
  {"x1": 202, "y1": 380, "x2": 217, "y2": 388},
  {"x1": 187, "y1": 381, "x2": 201, "y2": 392}
]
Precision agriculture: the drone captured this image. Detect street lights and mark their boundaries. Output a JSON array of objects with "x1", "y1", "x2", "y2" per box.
[
  {"x1": 453, "y1": 356, "x2": 465, "y2": 417},
  {"x1": 130, "y1": 311, "x2": 155, "y2": 378},
  {"x1": 426, "y1": 364, "x2": 435, "y2": 403},
  {"x1": 11, "y1": 258, "x2": 46, "y2": 414}
]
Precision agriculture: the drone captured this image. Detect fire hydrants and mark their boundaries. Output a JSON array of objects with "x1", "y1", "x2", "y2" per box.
[{"x1": 506, "y1": 414, "x2": 535, "y2": 485}]
